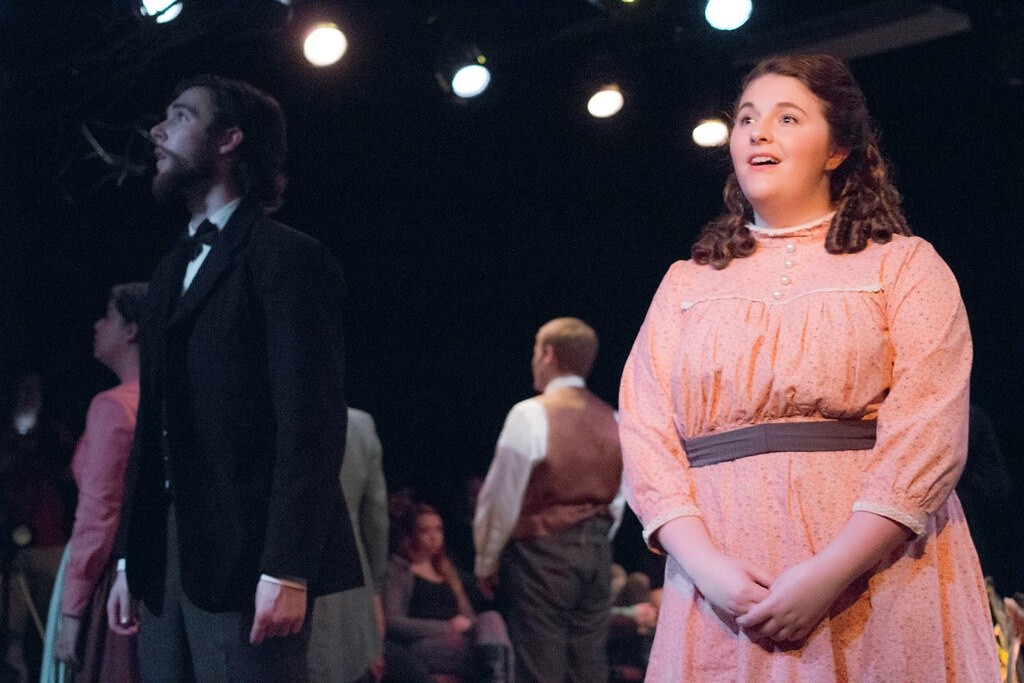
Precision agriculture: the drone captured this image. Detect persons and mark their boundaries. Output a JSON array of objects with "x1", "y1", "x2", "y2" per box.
[
  {"x1": 986, "y1": 592, "x2": 1024, "y2": 683},
  {"x1": 617, "y1": 54, "x2": 1002, "y2": 683},
  {"x1": 0, "y1": 278, "x2": 669, "y2": 683},
  {"x1": 108, "y1": 76, "x2": 351, "y2": 683}
]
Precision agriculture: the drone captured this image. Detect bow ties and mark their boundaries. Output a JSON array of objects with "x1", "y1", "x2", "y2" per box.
[{"x1": 173, "y1": 218, "x2": 220, "y2": 261}]
